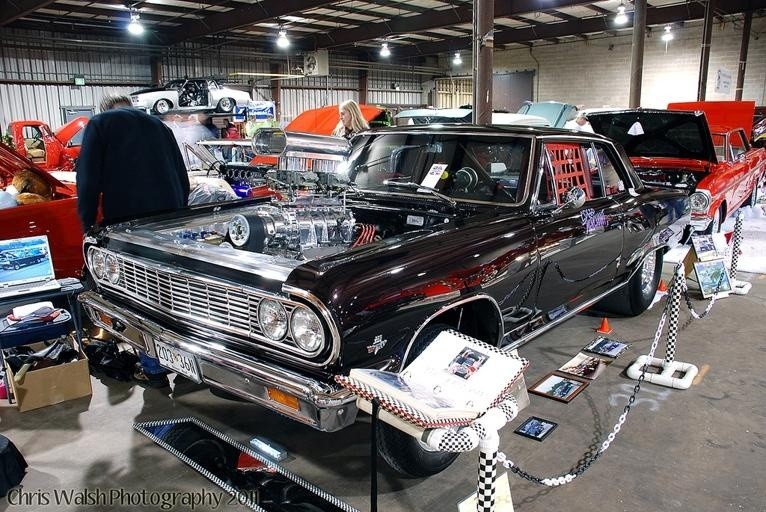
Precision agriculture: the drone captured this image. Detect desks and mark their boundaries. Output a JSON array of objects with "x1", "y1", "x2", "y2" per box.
[{"x1": 0, "y1": 278, "x2": 85, "y2": 405}]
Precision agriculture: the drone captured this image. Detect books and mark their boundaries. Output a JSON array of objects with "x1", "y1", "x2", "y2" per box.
[{"x1": 334, "y1": 329, "x2": 530, "y2": 429}]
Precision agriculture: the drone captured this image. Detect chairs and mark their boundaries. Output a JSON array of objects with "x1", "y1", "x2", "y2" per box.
[{"x1": 24, "y1": 140, "x2": 45, "y2": 162}]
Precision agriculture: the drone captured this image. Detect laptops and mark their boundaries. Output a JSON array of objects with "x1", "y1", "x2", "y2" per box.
[{"x1": 0, "y1": 235, "x2": 61, "y2": 298}]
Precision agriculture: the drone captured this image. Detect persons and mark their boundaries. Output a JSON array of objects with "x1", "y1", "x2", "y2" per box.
[
  {"x1": 76, "y1": 93, "x2": 191, "y2": 390},
  {"x1": 331, "y1": 100, "x2": 370, "y2": 138},
  {"x1": 564, "y1": 111, "x2": 595, "y2": 133}
]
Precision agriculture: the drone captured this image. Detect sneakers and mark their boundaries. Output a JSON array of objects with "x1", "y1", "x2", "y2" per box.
[{"x1": 133, "y1": 372, "x2": 169, "y2": 389}]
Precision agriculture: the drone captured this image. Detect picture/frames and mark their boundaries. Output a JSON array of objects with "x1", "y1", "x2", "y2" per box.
[
  {"x1": 694, "y1": 260, "x2": 736, "y2": 299},
  {"x1": 691, "y1": 233, "x2": 719, "y2": 259},
  {"x1": 527, "y1": 371, "x2": 590, "y2": 405},
  {"x1": 513, "y1": 416, "x2": 560, "y2": 442},
  {"x1": 584, "y1": 336, "x2": 633, "y2": 358}
]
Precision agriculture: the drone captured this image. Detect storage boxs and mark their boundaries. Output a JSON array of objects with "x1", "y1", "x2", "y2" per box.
[{"x1": 5, "y1": 335, "x2": 92, "y2": 413}]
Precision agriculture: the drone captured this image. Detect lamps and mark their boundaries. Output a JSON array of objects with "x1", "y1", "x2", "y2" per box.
[
  {"x1": 127, "y1": 6, "x2": 144, "y2": 35},
  {"x1": 453, "y1": 52, "x2": 462, "y2": 65},
  {"x1": 74, "y1": 74, "x2": 85, "y2": 86},
  {"x1": 380, "y1": 38, "x2": 391, "y2": 57},
  {"x1": 276, "y1": 30, "x2": 290, "y2": 49},
  {"x1": 614, "y1": 0, "x2": 629, "y2": 25},
  {"x1": 661, "y1": 23, "x2": 674, "y2": 41}
]
